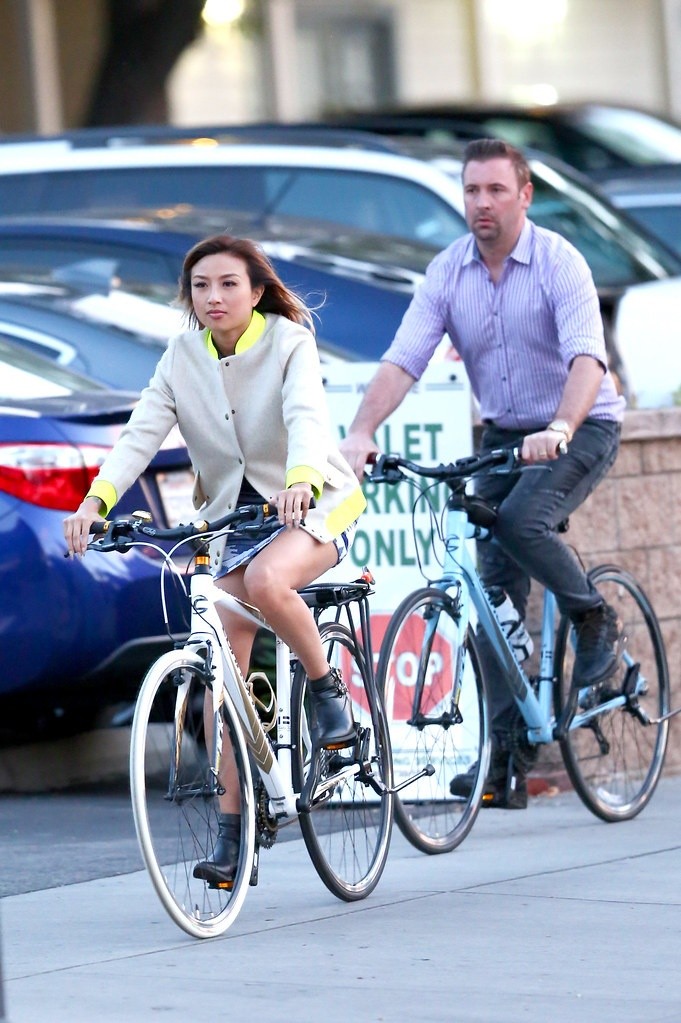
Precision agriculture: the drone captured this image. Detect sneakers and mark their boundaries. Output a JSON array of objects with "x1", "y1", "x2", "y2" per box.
[
  {"x1": 450, "y1": 749, "x2": 527, "y2": 809},
  {"x1": 568, "y1": 598, "x2": 626, "y2": 686}
]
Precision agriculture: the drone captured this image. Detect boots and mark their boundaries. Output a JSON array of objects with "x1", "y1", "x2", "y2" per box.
[
  {"x1": 193, "y1": 813, "x2": 259, "y2": 886},
  {"x1": 308, "y1": 667, "x2": 357, "y2": 745}
]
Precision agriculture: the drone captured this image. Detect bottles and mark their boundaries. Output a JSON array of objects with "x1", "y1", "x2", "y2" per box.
[{"x1": 486, "y1": 586, "x2": 534, "y2": 662}]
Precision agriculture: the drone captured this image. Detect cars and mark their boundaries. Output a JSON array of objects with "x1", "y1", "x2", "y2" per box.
[{"x1": 2, "y1": 96, "x2": 681, "y2": 739}]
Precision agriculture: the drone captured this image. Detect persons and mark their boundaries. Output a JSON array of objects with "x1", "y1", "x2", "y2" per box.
[
  {"x1": 339, "y1": 140, "x2": 626, "y2": 812},
  {"x1": 62, "y1": 233, "x2": 369, "y2": 882}
]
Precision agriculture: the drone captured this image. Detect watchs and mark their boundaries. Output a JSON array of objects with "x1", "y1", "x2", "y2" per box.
[{"x1": 548, "y1": 420, "x2": 572, "y2": 443}]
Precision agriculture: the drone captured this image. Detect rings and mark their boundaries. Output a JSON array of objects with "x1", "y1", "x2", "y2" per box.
[{"x1": 540, "y1": 452, "x2": 546, "y2": 456}]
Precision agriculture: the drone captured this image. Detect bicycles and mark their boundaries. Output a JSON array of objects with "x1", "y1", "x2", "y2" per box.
[
  {"x1": 64, "y1": 495, "x2": 438, "y2": 939},
  {"x1": 361, "y1": 436, "x2": 681, "y2": 856}
]
